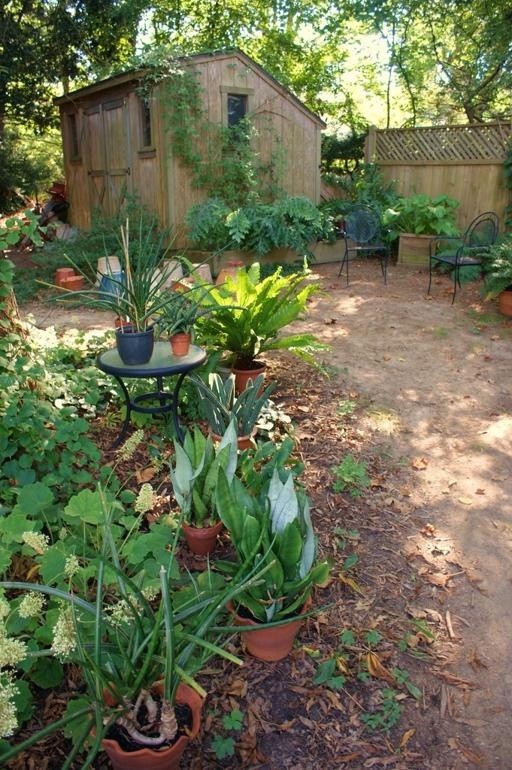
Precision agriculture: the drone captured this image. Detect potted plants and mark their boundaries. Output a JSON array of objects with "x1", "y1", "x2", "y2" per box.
[
  {"x1": 381, "y1": 192, "x2": 462, "y2": 270},
  {"x1": 34, "y1": 214, "x2": 216, "y2": 367},
  {"x1": 179, "y1": 262, "x2": 331, "y2": 396},
  {"x1": 216, "y1": 439, "x2": 329, "y2": 660},
  {"x1": 472, "y1": 240, "x2": 511, "y2": 318},
  {"x1": 148, "y1": 282, "x2": 249, "y2": 359},
  {"x1": 168, "y1": 415, "x2": 238, "y2": 555},
  {"x1": 184, "y1": 372, "x2": 277, "y2": 451},
  {"x1": 2, "y1": 501, "x2": 330, "y2": 769}
]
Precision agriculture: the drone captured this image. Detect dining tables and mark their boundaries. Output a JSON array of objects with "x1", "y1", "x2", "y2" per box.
[
  {"x1": 472, "y1": 240, "x2": 511, "y2": 318},
  {"x1": 381, "y1": 192, "x2": 462, "y2": 270}
]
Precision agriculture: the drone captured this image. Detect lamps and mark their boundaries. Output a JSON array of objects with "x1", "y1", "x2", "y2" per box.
[
  {"x1": 426, "y1": 211, "x2": 499, "y2": 306},
  {"x1": 337, "y1": 205, "x2": 392, "y2": 288}
]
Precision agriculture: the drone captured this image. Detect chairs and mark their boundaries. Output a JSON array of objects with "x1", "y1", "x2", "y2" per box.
[
  {"x1": 337, "y1": 205, "x2": 392, "y2": 288},
  {"x1": 426, "y1": 211, "x2": 499, "y2": 306}
]
